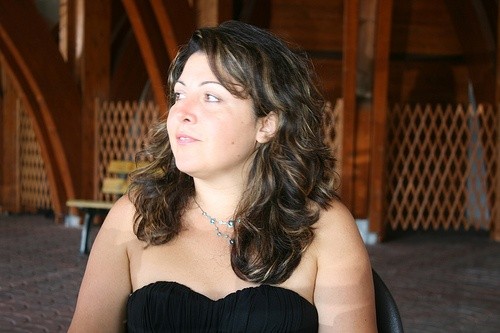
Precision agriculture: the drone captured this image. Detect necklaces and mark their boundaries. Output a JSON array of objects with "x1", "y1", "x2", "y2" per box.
[{"x1": 193, "y1": 198, "x2": 241, "y2": 245}]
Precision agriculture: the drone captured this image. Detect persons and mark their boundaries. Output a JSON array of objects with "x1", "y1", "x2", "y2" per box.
[{"x1": 66, "y1": 21, "x2": 378, "y2": 333}]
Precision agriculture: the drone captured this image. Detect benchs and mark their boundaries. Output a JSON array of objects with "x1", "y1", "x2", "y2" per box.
[{"x1": 66, "y1": 161, "x2": 164, "y2": 254}]
